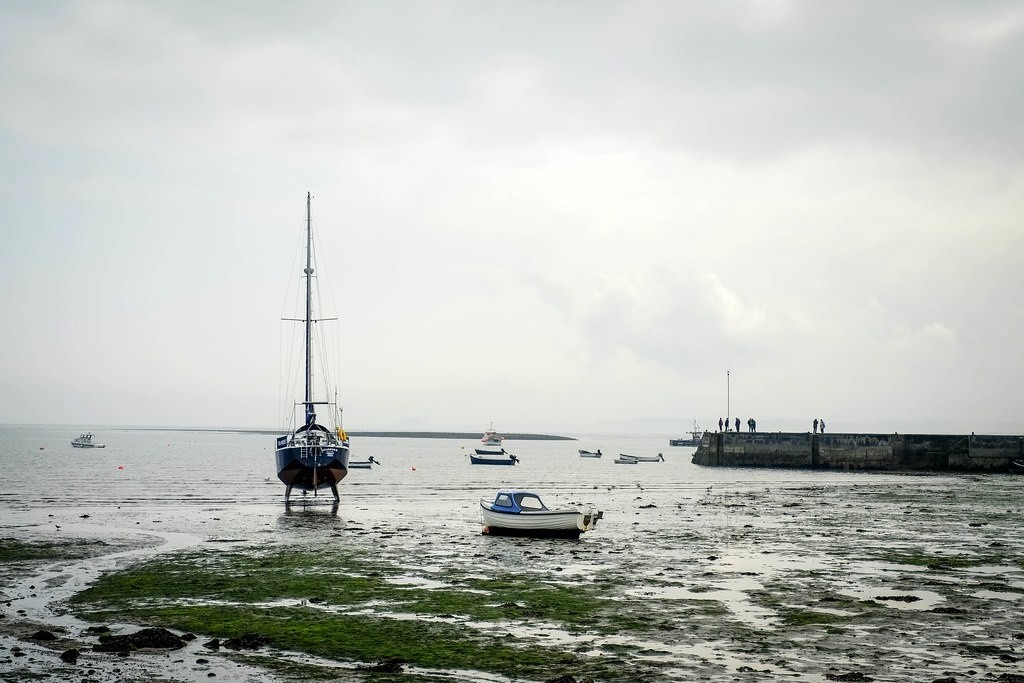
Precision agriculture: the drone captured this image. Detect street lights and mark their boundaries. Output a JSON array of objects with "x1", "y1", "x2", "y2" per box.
[{"x1": 726, "y1": 370, "x2": 731, "y2": 432}]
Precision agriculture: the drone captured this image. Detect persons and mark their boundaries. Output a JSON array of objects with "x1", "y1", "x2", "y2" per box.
[
  {"x1": 719, "y1": 418, "x2": 723, "y2": 430},
  {"x1": 725, "y1": 418, "x2": 729, "y2": 431},
  {"x1": 813, "y1": 418, "x2": 818, "y2": 434},
  {"x1": 735, "y1": 418, "x2": 740, "y2": 432},
  {"x1": 748, "y1": 418, "x2": 756, "y2": 433},
  {"x1": 820, "y1": 419, "x2": 825, "y2": 433}
]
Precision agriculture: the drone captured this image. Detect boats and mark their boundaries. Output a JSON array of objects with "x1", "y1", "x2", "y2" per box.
[
  {"x1": 475, "y1": 448, "x2": 506, "y2": 455},
  {"x1": 70, "y1": 432, "x2": 106, "y2": 448},
  {"x1": 348, "y1": 456, "x2": 380, "y2": 468},
  {"x1": 670, "y1": 419, "x2": 704, "y2": 446},
  {"x1": 484, "y1": 421, "x2": 504, "y2": 446},
  {"x1": 479, "y1": 490, "x2": 605, "y2": 537},
  {"x1": 578, "y1": 449, "x2": 602, "y2": 458},
  {"x1": 614, "y1": 458, "x2": 640, "y2": 465},
  {"x1": 620, "y1": 453, "x2": 663, "y2": 462},
  {"x1": 469, "y1": 453, "x2": 519, "y2": 465}
]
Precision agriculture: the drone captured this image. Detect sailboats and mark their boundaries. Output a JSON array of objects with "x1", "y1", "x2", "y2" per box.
[{"x1": 272, "y1": 188, "x2": 350, "y2": 515}]
